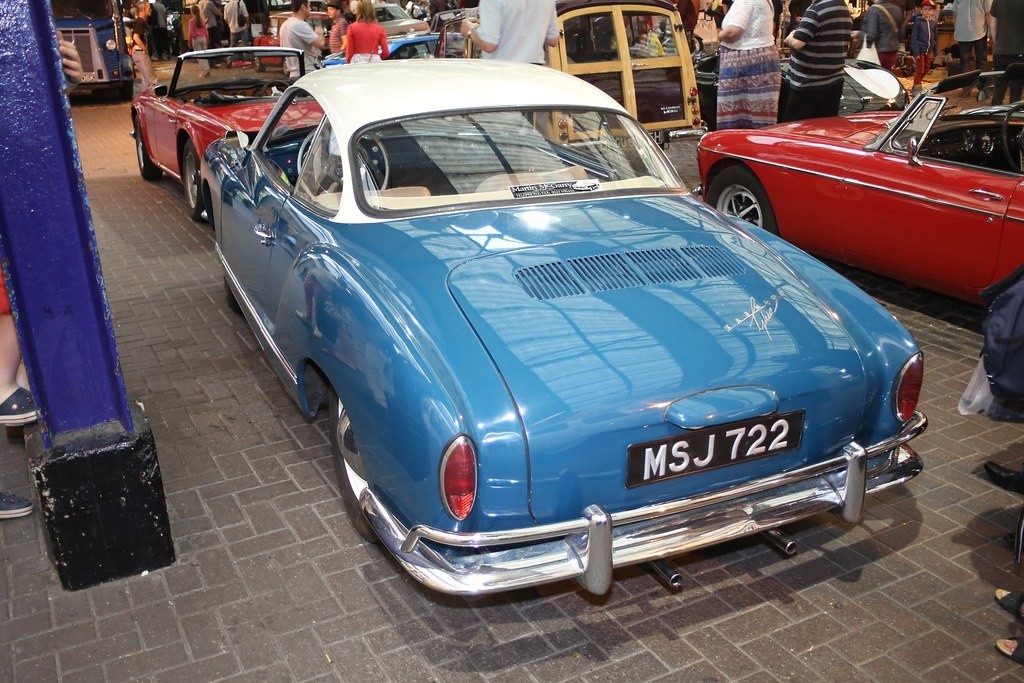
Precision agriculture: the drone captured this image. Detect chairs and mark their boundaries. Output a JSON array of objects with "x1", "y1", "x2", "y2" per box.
[
  {"x1": 313, "y1": 186, "x2": 431, "y2": 209},
  {"x1": 474, "y1": 165, "x2": 589, "y2": 193}
]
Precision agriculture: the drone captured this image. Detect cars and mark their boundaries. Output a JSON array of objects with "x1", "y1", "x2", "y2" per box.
[
  {"x1": 253, "y1": 12, "x2": 330, "y2": 77},
  {"x1": 465, "y1": 0, "x2": 702, "y2": 153},
  {"x1": 321, "y1": 2, "x2": 479, "y2": 67},
  {"x1": 695, "y1": 63, "x2": 1024, "y2": 307},
  {"x1": 692, "y1": 52, "x2": 909, "y2": 132},
  {"x1": 200, "y1": 59, "x2": 928, "y2": 598},
  {"x1": 130, "y1": 46, "x2": 324, "y2": 223}
]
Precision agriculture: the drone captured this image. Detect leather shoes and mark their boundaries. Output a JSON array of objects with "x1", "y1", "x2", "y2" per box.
[{"x1": 983, "y1": 460, "x2": 1024, "y2": 494}]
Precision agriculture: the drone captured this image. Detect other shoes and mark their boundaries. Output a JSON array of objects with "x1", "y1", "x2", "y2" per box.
[
  {"x1": 210, "y1": 63, "x2": 223, "y2": 68},
  {"x1": 225, "y1": 60, "x2": 232, "y2": 69}
]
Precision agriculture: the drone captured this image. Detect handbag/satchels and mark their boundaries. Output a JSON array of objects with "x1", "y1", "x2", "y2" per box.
[
  {"x1": 238, "y1": 0, "x2": 245, "y2": 27},
  {"x1": 856, "y1": 36, "x2": 882, "y2": 66}
]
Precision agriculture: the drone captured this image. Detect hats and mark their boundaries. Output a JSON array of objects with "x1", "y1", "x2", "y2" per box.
[
  {"x1": 325, "y1": 0, "x2": 344, "y2": 13},
  {"x1": 920, "y1": 0, "x2": 936, "y2": 10}
]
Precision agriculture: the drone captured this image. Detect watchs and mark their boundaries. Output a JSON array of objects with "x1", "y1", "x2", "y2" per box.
[{"x1": 468, "y1": 28, "x2": 477, "y2": 39}]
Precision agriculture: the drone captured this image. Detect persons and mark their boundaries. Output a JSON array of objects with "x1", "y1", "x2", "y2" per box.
[
  {"x1": 952, "y1": 0, "x2": 996, "y2": 98},
  {"x1": 371, "y1": 0, "x2": 386, "y2": 4},
  {"x1": 718, "y1": 0, "x2": 781, "y2": 131},
  {"x1": 991, "y1": 0, "x2": 1024, "y2": 105},
  {"x1": 705, "y1": 0, "x2": 733, "y2": 28},
  {"x1": 280, "y1": 0, "x2": 325, "y2": 81},
  {"x1": 425, "y1": 0, "x2": 445, "y2": 19},
  {"x1": 911, "y1": 0, "x2": 939, "y2": 96},
  {"x1": 774, "y1": 0, "x2": 853, "y2": 123},
  {"x1": 326, "y1": 3, "x2": 348, "y2": 54},
  {"x1": 133, "y1": 1, "x2": 153, "y2": 89},
  {"x1": 198, "y1": 0, "x2": 249, "y2": 67},
  {"x1": 122, "y1": 0, "x2": 171, "y2": 60},
  {"x1": 346, "y1": 0, "x2": 388, "y2": 64},
  {"x1": 850, "y1": 0, "x2": 916, "y2": 68},
  {"x1": 460, "y1": 0, "x2": 559, "y2": 65},
  {"x1": 0, "y1": 30, "x2": 83, "y2": 520},
  {"x1": 188, "y1": 6, "x2": 210, "y2": 78}
]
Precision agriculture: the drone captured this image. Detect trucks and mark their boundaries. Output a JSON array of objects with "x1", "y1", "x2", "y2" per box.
[{"x1": 51, "y1": 0, "x2": 136, "y2": 99}]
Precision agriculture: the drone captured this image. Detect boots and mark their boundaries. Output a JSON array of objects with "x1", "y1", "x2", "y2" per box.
[{"x1": 913, "y1": 85, "x2": 922, "y2": 97}]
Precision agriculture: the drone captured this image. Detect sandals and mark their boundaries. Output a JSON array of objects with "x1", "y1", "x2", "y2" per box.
[
  {"x1": 0, "y1": 490, "x2": 34, "y2": 520},
  {"x1": 995, "y1": 589, "x2": 1024, "y2": 618},
  {"x1": 996, "y1": 636, "x2": 1024, "y2": 664},
  {"x1": 0, "y1": 387, "x2": 38, "y2": 426}
]
